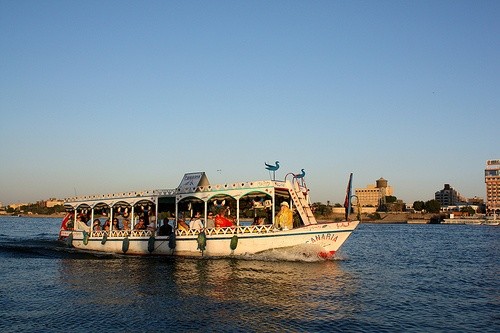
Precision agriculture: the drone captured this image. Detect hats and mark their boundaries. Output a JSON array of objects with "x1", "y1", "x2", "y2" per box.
[
  {"x1": 281, "y1": 202, "x2": 289, "y2": 208},
  {"x1": 151, "y1": 216, "x2": 159, "y2": 221}
]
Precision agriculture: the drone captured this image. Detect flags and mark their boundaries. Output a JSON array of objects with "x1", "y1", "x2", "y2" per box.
[{"x1": 344, "y1": 172, "x2": 352, "y2": 217}]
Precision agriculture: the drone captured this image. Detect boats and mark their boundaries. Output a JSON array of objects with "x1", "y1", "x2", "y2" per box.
[
  {"x1": 55, "y1": 160, "x2": 361, "y2": 261},
  {"x1": 482, "y1": 210, "x2": 500, "y2": 226},
  {"x1": 464, "y1": 219, "x2": 482, "y2": 226}
]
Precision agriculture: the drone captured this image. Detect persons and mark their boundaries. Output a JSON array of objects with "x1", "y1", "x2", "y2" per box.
[
  {"x1": 174, "y1": 215, "x2": 190, "y2": 232},
  {"x1": 112, "y1": 218, "x2": 121, "y2": 235},
  {"x1": 276, "y1": 202, "x2": 295, "y2": 230},
  {"x1": 157, "y1": 218, "x2": 173, "y2": 235},
  {"x1": 214, "y1": 207, "x2": 237, "y2": 234},
  {"x1": 136, "y1": 218, "x2": 145, "y2": 233},
  {"x1": 76, "y1": 214, "x2": 110, "y2": 232},
  {"x1": 204, "y1": 211, "x2": 215, "y2": 231},
  {"x1": 189, "y1": 212, "x2": 204, "y2": 232},
  {"x1": 249, "y1": 215, "x2": 267, "y2": 233},
  {"x1": 147, "y1": 217, "x2": 157, "y2": 234}
]
